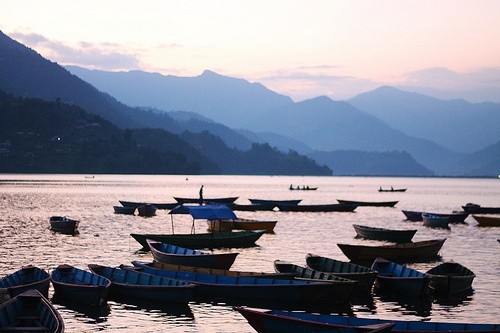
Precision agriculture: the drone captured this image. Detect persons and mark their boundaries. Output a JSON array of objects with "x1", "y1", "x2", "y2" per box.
[{"x1": 200, "y1": 185, "x2": 204, "y2": 198}]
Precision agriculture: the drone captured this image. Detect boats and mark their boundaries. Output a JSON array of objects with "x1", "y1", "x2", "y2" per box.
[
  {"x1": 49, "y1": 264, "x2": 112, "y2": 307},
  {"x1": 49, "y1": 216, "x2": 80, "y2": 236},
  {"x1": 371, "y1": 257, "x2": 429, "y2": 297},
  {"x1": 129, "y1": 204, "x2": 267, "y2": 252},
  {"x1": 304, "y1": 251, "x2": 378, "y2": 292},
  {"x1": 117, "y1": 200, "x2": 359, "y2": 212},
  {"x1": 337, "y1": 237, "x2": 448, "y2": 262},
  {"x1": 378, "y1": 188, "x2": 408, "y2": 192},
  {"x1": 249, "y1": 198, "x2": 302, "y2": 204},
  {"x1": 426, "y1": 260, "x2": 473, "y2": 295},
  {"x1": 401, "y1": 203, "x2": 500, "y2": 228},
  {"x1": 232, "y1": 305, "x2": 500, "y2": 333},
  {"x1": 0, "y1": 264, "x2": 52, "y2": 304},
  {"x1": 145, "y1": 238, "x2": 240, "y2": 271},
  {"x1": 207, "y1": 217, "x2": 278, "y2": 234},
  {"x1": 289, "y1": 186, "x2": 318, "y2": 190},
  {"x1": 351, "y1": 223, "x2": 417, "y2": 243},
  {"x1": 87, "y1": 263, "x2": 198, "y2": 309},
  {"x1": 0, "y1": 288, "x2": 65, "y2": 333},
  {"x1": 273, "y1": 258, "x2": 359, "y2": 307},
  {"x1": 173, "y1": 196, "x2": 240, "y2": 204},
  {"x1": 119, "y1": 259, "x2": 361, "y2": 310},
  {"x1": 337, "y1": 199, "x2": 400, "y2": 207},
  {"x1": 114, "y1": 205, "x2": 156, "y2": 217}
]
